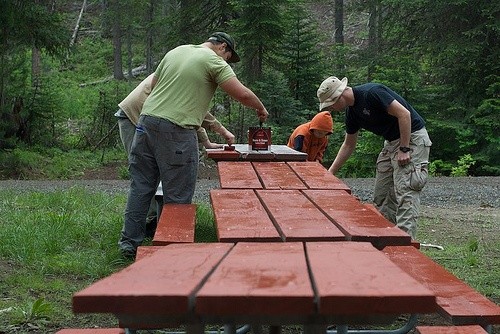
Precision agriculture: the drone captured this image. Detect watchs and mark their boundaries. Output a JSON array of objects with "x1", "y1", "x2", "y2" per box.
[{"x1": 399, "y1": 146, "x2": 411, "y2": 152}]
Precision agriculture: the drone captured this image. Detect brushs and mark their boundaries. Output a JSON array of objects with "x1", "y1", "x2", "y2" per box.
[
  {"x1": 224, "y1": 143, "x2": 235, "y2": 151},
  {"x1": 251, "y1": 121, "x2": 269, "y2": 142}
]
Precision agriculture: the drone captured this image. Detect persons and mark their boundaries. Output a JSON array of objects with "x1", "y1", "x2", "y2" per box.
[
  {"x1": 114, "y1": 72, "x2": 235, "y2": 225},
  {"x1": 287, "y1": 111, "x2": 333, "y2": 162},
  {"x1": 118, "y1": 32, "x2": 269, "y2": 256},
  {"x1": 317, "y1": 75, "x2": 432, "y2": 241}
]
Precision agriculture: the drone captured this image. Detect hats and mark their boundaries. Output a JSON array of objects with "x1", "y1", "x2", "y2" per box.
[
  {"x1": 317, "y1": 76, "x2": 348, "y2": 111},
  {"x1": 207, "y1": 31, "x2": 240, "y2": 64}
]
